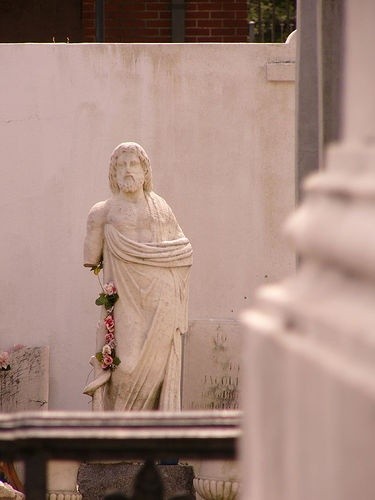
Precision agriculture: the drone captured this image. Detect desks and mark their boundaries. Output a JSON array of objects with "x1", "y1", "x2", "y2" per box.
[{"x1": 0, "y1": 409, "x2": 240, "y2": 500}]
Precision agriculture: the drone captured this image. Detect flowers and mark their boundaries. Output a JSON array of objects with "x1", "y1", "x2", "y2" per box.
[{"x1": 92, "y1": 263, "x2": 122, "y2": 371}]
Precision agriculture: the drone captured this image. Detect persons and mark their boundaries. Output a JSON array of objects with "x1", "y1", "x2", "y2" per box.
[{"x1": 85, "y1": 141, "x2": 194, "y2": 414}]
